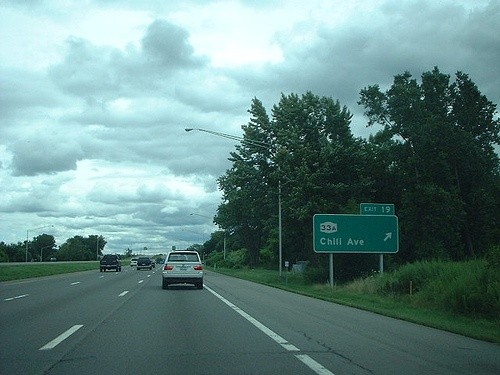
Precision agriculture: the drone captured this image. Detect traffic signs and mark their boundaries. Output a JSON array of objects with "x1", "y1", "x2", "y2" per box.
[{"x1": 314, "y1": 215, "x2": 400, "y2": 253}]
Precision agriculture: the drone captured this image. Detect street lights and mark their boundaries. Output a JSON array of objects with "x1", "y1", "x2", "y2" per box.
[
  {"x1": 182, "y1": 229, "x2": 204, "y2": 263},
  {"x1": 190, "y1": 213, "x2": 226, "y2": 260},
  {"x1": 25, "y1": 225, "x2": 54, "y2": 262},
  {"x1": 186, "y1": 127, "x2": 282, "y2": 277}
]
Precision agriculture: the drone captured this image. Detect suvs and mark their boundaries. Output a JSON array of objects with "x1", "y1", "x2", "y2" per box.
[
  {"x1": 150, "y1": 258, "x2": 156, "y2": 268},
  {"x1": 137, "y1": 257, "x2": 152, "y2": 270},
  {"x1": 100, "y1": 255, "x2": 122, "y2": 272},
  {"x1": 130, "y1": 258, "x2": 139, "y2": 267},
  {"x1": 162, "y1": 250, "x2": 204, "y2": 289}
]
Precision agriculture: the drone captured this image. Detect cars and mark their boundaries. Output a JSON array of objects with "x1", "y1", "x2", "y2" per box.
[{"x1": 156, "y1": 258, "x2": 164, "y2": 264}]
